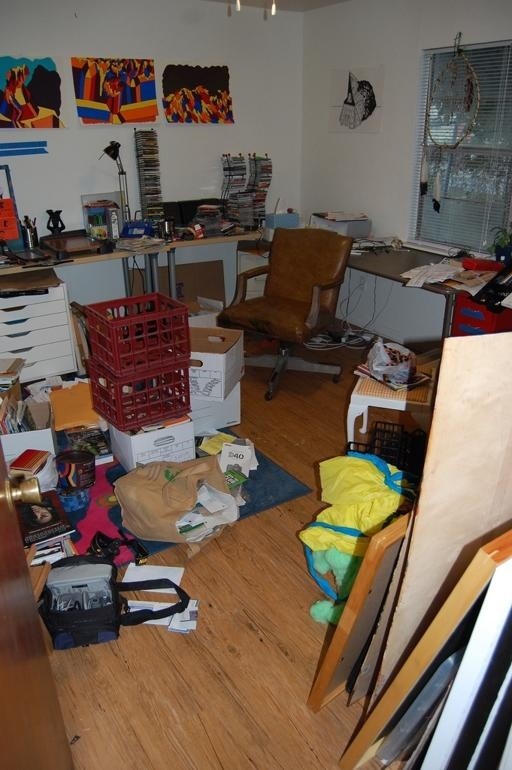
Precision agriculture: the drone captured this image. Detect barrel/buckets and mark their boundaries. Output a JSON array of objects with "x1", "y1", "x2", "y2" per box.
[{"x1": 55, "y1": 451, "x2": 96, "y2": 490}]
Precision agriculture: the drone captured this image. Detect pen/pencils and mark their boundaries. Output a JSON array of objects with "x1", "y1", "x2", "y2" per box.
[{"x1": 21, "y1": 215, "x2": 37, "y2": 229}]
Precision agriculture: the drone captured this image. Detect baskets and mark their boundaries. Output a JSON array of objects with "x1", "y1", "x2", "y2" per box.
[
  {"x1": 346, "y1": 420, "x2": 429, "y2": 475},
  {"x1": 86, "y1": 357, "x2": 192, "y2": 433},
  {"x1": 85, "y1": 289, "x2": 191, "y2": 379}
]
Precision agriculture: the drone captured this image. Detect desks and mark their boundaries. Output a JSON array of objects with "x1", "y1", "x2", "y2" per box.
[
  {"x1": 236, "y1": 240, "x2": 465, "y2": 343},
  {"x1": 1, "y1": 225, "x2": 263, "y2": 301}
]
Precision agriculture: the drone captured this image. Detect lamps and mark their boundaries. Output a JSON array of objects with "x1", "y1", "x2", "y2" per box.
[{"x1": 103, "y1": 140, "x2": 131, "y2": 226}]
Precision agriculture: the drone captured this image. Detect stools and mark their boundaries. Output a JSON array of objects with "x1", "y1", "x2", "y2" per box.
[{"x1": 345, "y1": 365, "x2": 438, "y2": 451}]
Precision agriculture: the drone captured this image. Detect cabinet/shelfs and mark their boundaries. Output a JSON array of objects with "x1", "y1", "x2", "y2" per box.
[{"x1": 0, "y1": 268, "x2": 78, "y2": 383}]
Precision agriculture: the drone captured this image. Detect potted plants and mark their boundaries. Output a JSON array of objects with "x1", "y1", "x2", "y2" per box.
[{"x1": 486, "y1": 223, "x2": 511, "y2": 264}]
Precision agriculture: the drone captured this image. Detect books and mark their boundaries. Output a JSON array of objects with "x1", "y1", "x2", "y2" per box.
[
  {"x1": 11, "y1": 448, "x2": 51, "y2": 475},
  {"x1": 15, "y1": 490, "x2": 80, "y2": 567},
  {"x1": 68, "y1": 427, "x2": 113, "y2": 466},
  {"x1": 353, "y1": 363, "x2": 431, "y2": 391}
]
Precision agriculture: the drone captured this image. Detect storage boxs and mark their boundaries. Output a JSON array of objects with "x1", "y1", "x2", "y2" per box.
[{"x1": 108, "y1": 295, "x2": 247, "y2": 474}]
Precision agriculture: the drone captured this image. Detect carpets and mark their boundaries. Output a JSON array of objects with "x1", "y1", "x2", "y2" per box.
[{"x1": 68, "y1": 426, "x2": 314, "y2": 570}]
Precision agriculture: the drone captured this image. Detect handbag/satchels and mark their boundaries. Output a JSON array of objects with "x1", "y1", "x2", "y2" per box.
[
  {"x1": 41, "y1": 554, "x2": 123, "y2": 649},
  {"x1": 113, "y1": 456, "x2": 231, "y2": 559}
]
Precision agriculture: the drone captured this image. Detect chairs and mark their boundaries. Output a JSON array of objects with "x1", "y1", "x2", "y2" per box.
[{"x1": 215, "y1": 227, "x2": 354, "y2": 400}]
